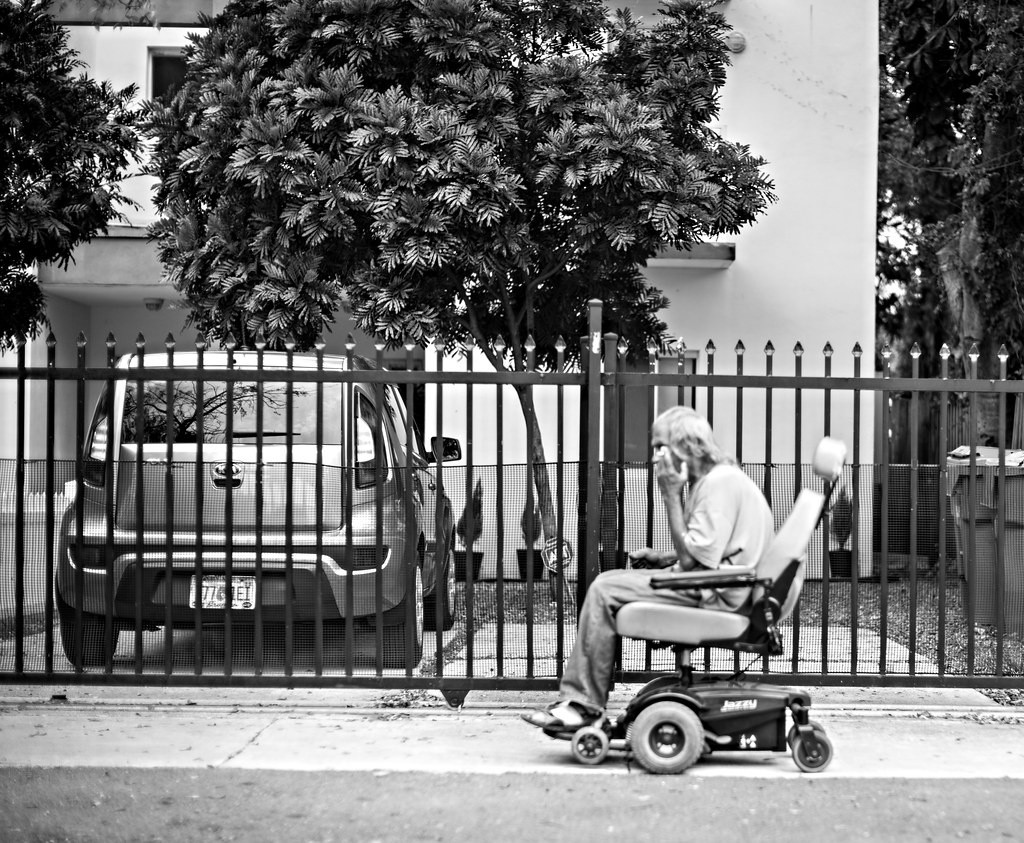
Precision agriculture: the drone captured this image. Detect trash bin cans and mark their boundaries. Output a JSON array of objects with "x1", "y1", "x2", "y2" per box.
[{"x1": 948, "y1": 445, "x2": 1024, "y2": 635}]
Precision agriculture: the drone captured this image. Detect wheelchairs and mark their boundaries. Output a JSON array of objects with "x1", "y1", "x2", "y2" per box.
[{"x1": 539, "y1": 431, "x2": 849, "y2": 774}]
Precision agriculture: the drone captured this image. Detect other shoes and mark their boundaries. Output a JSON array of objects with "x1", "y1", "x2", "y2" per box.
[{"x1": 521, "y1": 702, "x2": 602, "y2": 730}]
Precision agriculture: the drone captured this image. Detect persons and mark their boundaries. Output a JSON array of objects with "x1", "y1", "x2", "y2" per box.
[{"x1": 517, "y1": 406, "x2": 777, "y2": 734}]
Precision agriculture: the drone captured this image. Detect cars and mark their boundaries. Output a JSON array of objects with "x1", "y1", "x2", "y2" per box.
[{"x1": 54, "y1": 344, "x2": 474, "y2": 668}]
